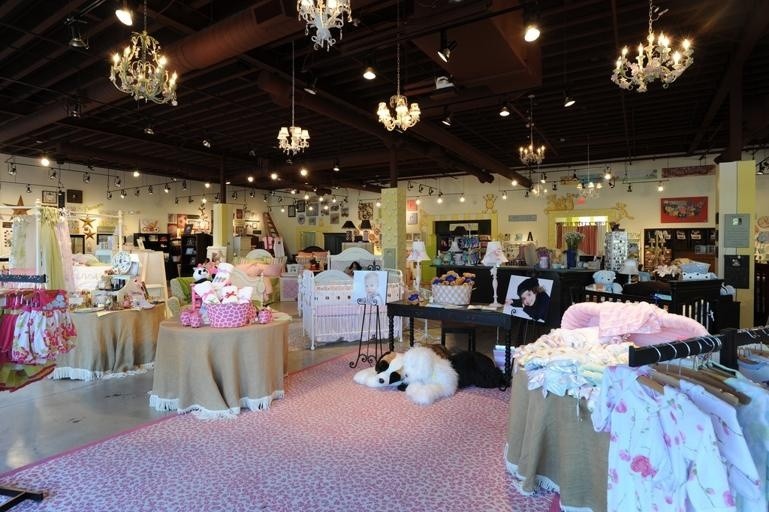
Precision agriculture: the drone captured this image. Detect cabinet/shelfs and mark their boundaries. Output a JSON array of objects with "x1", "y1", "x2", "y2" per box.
[{"x1": 133, "y1": 213, "x2": 213, "y2": 250}]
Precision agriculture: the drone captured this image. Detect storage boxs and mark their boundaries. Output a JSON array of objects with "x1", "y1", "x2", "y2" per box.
[{"x1": 430, "y1": 284, "x2": 473, "y2": 305}]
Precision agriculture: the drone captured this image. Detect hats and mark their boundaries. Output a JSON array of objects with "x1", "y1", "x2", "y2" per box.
[{"x1": 517, "y1": 278, "x2": 539, "y2": 296}]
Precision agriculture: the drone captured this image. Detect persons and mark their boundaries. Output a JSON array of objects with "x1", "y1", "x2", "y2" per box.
[
  {"x1": 505, "y1": 277, "x2": 549, "y2": 321},
  {"x1": 362, "y1": 273, "x2": 382, "y2": 304}
]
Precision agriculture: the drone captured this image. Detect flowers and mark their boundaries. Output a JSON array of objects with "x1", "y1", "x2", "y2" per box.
[
  {"x1": 534, "y1": 246, "x2": 552, "y2": 257},
  {"x1": 565, "y1": 230, "x2": 582, "y2": 251}
]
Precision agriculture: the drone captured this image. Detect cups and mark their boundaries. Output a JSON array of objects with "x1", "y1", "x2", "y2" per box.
[{"x1": 455, "y1": 254, "x2": 462, "y2": 264}]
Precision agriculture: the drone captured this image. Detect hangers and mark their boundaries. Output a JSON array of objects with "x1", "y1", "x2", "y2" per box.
[{"x1": 629, "y1": 325, "x2": 767, "y2": 410}]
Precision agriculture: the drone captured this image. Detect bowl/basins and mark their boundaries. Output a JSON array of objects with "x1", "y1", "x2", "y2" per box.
[{"x1": 552, "y1": 263, "x2": 561, "y2": 269}]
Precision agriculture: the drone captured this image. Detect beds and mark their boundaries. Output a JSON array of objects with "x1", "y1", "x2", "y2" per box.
[
  {"x1": 287, "y1": 241, "x2": 405, "y2": 349},
  {"x1": 573, "y1": 278, "x2": 738, "y2": 334}
]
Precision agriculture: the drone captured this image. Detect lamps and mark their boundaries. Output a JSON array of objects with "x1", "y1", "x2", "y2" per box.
[
  {"x1": 341, "y1": 220, "x2": 355, "y2": 241},
  {"x1": 441, "y1": 88, "x2": 576, "y2": 126},
  {"x1": 407, "y1": 242, "x2": 432, "y2": 300},
  {"x1": 519, "y1": 93, "x2": 547, "y2": 164},
  {"x1": 448, "y1": 241, "x2": 461, "y2": 264},
  {"x1": 277, "y1": 41, "x2": 310, "y2": 154},
  {"x1": 301, "y1": 4, "x2": 542, "y2": 96},
  {"x1": 295, "y1": 0, "x2": 352, "y2": 52},
  {"x1": 618, "y1": 259, "x2": 639, "y2": 288},
  {"x1": 64, "y1": 0, "x2": 132, "y2": 51},
  {"x1": 609, "y1": 0, "x2": 697, "y2": 94},
  {"x1": 360, "y1": 220, "x2": 371, "y2": 241},
  {"x1": 482, "y1": 240, "x2": 508, "y2": 307},
  {"x1": 108, "y1": 1, "x2": 179, "y2": 106},
  {"x1": 377, "y1": 0, "x2": 422, "y2": 131}
]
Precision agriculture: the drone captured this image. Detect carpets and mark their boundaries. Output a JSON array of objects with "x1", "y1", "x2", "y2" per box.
[{"x1": 0, "y1": 340, "x2": 613, "y2": 510}]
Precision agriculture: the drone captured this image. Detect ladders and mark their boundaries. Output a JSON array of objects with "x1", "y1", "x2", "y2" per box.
[{"x1": 263, "y1": 212, "x2": 288, "y2": 256}]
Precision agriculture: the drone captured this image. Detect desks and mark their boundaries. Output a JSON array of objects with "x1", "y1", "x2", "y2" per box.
[
  {"x1": 510, "y1": 335, "x2": 630, "y2": 510},
  {"x1": 387, "y1": 297, "x2": 525, "y2": 358}
]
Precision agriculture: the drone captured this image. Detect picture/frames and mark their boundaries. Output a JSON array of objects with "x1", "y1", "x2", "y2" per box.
[{"x1": 287, "y1": 205, "x2": 296, "y2": 218}]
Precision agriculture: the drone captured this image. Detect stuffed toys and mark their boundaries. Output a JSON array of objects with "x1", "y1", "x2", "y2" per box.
[
  {"x1": 350, "y1": 342, "x2": 449, "y2": 389},
  {"x1": 591, "y1": 269, "x2": 623, "y2": 295},
  {"x1": 191, "y1": 261, "x2": 254, "y2": 305},
  {"x1": 449, "y1": 349, "x2": 506, "y2": 390},
  {"x1": 651, "y1": 257, "x2": 689, "y2": 277},
  {"x1": 402, "y1": 345, "x2": 457, "y2": 403}
]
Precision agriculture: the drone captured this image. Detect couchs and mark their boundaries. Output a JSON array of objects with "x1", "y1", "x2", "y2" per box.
[{"x1": 641, "y1": 256, "x2": 718, "y2": 281}]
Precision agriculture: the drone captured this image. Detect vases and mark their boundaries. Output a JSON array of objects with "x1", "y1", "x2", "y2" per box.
[
  {"x1": 567, "y1": 251, "x2": 577, "y2": 268},
  {"x1": 539, "y1": 257, "x2": 547, "y2": 268}
]
